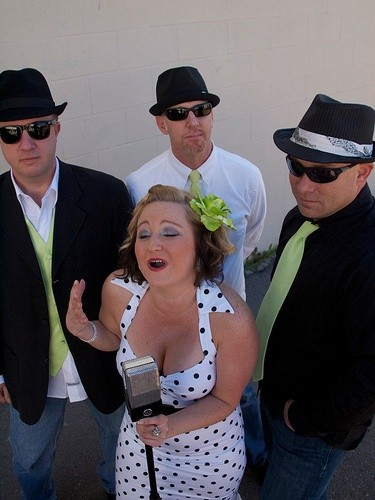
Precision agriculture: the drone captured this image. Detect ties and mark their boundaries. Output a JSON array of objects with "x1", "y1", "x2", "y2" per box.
[
  {"x1": 190, "y1": 170, "x2": 200, "y2": 196},
  {"x1": 252, "y1": 221, "x2": 320, "y2": 381}
]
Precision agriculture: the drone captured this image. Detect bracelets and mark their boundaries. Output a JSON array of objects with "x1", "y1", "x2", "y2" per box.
[{"x1": 78, "y1": 321, "x2": 97, "y2": 343}]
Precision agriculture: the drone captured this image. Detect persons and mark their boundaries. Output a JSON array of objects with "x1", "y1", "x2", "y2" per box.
[
  {"x1": 252, "y1": 93, "x2": 375, "y2": 500},
  {"x1": 66, "y1": 184, "x2": 258, "y2": 500},
  {"x1": 0, "y1": 67, "x2": 137, "y2": 500},
  {"x1": 125, "y1": 67, "x2": 267, "y2": 486}
]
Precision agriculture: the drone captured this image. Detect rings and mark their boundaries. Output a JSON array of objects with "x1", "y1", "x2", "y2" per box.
[{"x1": 152, "y1": 424, "x2": 161, "y2": 437}]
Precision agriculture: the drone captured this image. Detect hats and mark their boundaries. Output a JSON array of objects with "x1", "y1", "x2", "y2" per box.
[
  {"x1": 0, "y1": 68, "x2": 68, "y2": 122},
  {"x1": 273, "y1": 93, "x2": 375, "y2": 165},
  {"x1": 149, "y1": 65, "x2": 220, "y2": 115}
]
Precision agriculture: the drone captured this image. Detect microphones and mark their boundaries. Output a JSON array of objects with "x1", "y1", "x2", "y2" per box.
[{"x1": 121, "y1": 354, "x2": 162, "y2": 500}]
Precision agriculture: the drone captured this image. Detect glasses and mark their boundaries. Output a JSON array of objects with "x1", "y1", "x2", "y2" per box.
[
  {"x1": 0, "y1": 119, "x2": 58, "y2": 144},
  {"x1": 285, "y1": 155, "x2": 359, "y2": 184},
  {"x1": 165, "y1": 102, "x2": 213, "y2": 121}
]
